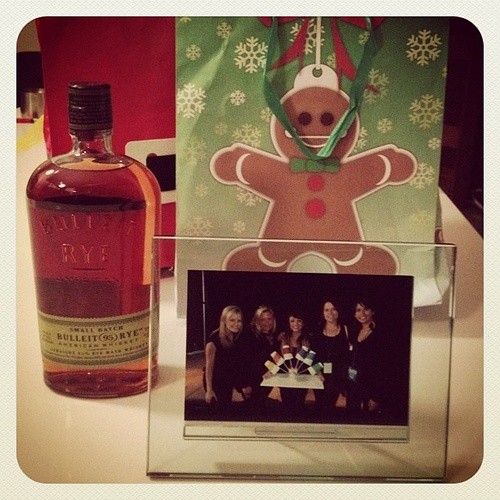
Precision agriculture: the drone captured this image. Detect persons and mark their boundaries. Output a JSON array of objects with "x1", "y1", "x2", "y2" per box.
[
  {"x1": 270, "y1": 309, "x2": 313, "y2": 409},
  {"x1": 233, "y1": 305, "x2": 279, "y2": 413},
  {"x1": 341, "y1": 298, "x2": 388, "y2": 407},
  {"x1": 279, "y1": 298, "x2": 351, "y2": 418},
  {"x1": 204, "y1": 306, "x2": 243, "y2": 415}
]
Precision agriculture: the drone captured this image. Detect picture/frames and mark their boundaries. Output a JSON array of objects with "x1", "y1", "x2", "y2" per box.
[{"x1": 143, "y1": 232, "x2": 459, "y2": 485}]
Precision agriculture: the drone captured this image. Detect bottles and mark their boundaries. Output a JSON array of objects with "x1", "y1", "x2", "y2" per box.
[{"x1": 27, "y1": 81, "x2": 163, "y2": 399}]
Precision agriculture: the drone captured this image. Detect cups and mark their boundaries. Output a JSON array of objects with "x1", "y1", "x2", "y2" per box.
[
  {"x1": 295, "y1": 345, "x2": 309, "y2": 361},
  {"x1": 270, "y1": 351, "x2": 285, "y2": 366},
  {"x1": 302, "y1": 350, "x2": 316, "y2": 366},
  {"x1": 264, "y1": 360, "x2": 280, "y2": 374},
  {"x1": 307, "y1": 362, "x2": 323, "y2": 376},
  {"x1": 282, "y1": 345, "x2": 293, "y2": 360}
]
